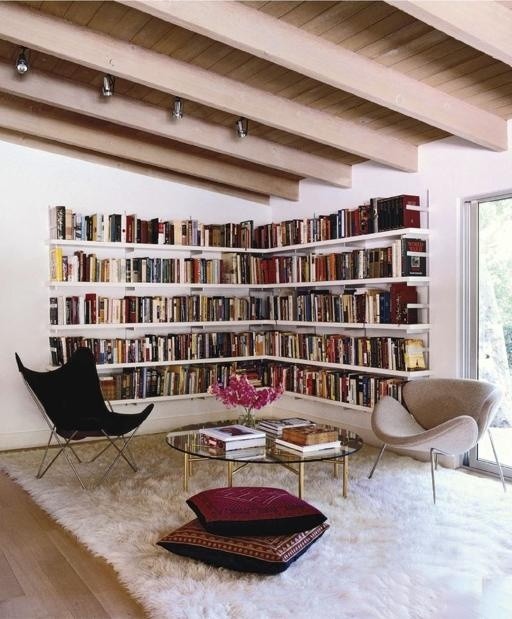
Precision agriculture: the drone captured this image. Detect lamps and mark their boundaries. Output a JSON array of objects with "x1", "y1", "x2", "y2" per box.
[
  {"x1": 170, "y1": 95, "x2": 250, "y2": 139},
  {"x1": 14, "y1": 46, "x2": 117, "y2": 100}
]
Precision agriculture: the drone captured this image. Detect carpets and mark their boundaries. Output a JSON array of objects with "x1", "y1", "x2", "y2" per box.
[{"x1": 1, "y1": 427, "x2": 511, "y2": 619}]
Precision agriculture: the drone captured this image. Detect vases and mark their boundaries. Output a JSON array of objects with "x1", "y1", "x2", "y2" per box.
[{"x1": 239, "y1": 407, "x2": 256, "y2": 428}]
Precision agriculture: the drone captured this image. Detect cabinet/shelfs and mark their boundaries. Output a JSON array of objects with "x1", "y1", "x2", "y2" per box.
[
  {"x1": 50, "y1": 206, "x2": 266, "y2": 404},
  {"x1": 267, "y1": 204, "x2": 430, "y2": 410}
]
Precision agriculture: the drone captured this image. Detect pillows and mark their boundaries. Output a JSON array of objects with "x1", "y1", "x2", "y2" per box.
[{"x1": 154, "y1": 485, "x2": 330, "y2": 579}]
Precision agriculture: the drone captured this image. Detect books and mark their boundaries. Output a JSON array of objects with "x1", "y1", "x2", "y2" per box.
[
  {"x1": 198, "y1": 416, "x2": 342, "y2": 454},
  {"x1": 200, "y1": 445, "x2": 341, "y2": 461}
]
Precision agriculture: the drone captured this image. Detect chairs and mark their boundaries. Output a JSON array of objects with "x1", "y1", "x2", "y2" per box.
[
  {"x1": 12, "y1": 345, "x2": 156, "y2": 493},
  {"x1": 365, "y1": 376, "x2": 511, "y2": 509}
]
